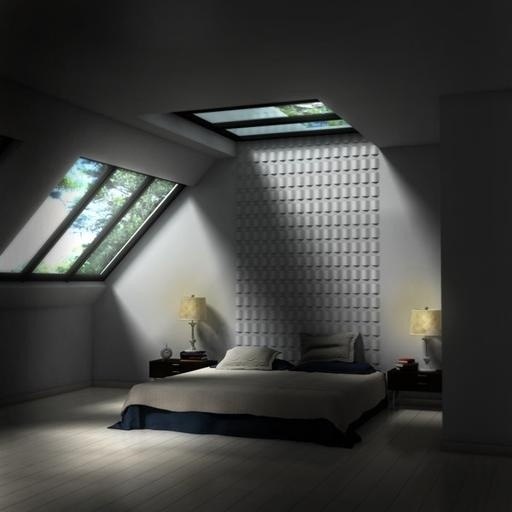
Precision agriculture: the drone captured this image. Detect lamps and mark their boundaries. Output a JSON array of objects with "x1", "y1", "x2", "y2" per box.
[
  {"x1": 408, "y1": 305, "x2": 441, "y2": 372},
  {"x1": 176, "y1": 294, "x2": 210, "y2": 359}
]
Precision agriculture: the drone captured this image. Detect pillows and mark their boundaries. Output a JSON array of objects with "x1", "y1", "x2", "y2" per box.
[{"x1": 216, "y1": 332, "x2": 376, "y2": 375}]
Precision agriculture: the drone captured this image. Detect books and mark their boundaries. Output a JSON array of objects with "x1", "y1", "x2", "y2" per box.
[
  {"x1": 393, "y1": 357, "x2": 419, "y2": 371},
  {"x1": 180, "y1": 349, "x2": 208, "y2": 362}
]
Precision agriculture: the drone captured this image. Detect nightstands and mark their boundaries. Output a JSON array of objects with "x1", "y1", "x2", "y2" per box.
[
  {"x1": 149, "y1": 358, "x2": 218, "y2": 383},
  {"x1": 387, "y1": 365, "x2": 442, "y2": 409}
]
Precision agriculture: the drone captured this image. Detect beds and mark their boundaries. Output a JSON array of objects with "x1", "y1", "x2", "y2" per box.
[{"x1": 121, "y1": 365, "x2": 386, "y2": 449}]
